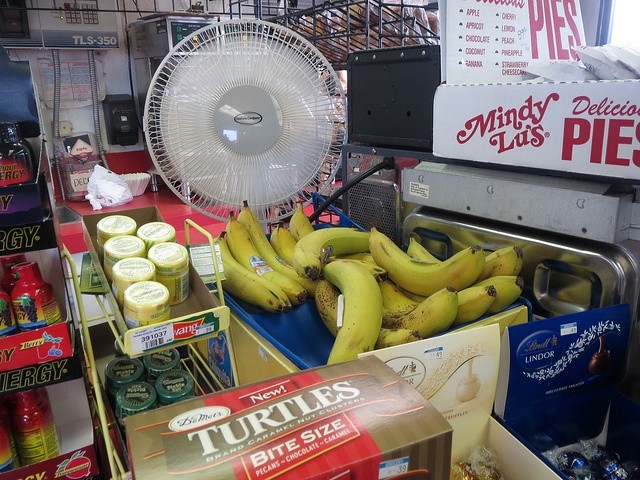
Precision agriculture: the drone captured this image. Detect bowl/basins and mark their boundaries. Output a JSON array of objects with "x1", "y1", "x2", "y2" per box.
[{"x1": 117, "y1": 172, "x2": 151, "y2": 197}]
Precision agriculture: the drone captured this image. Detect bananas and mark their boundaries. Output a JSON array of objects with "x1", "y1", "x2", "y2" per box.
[{"x1": 214, "y1": 200, "x2": 522, "y2": 367}]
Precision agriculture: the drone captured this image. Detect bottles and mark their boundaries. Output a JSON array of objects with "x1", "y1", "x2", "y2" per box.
[
  {"x1": 113, "y1": 336, "x2": 126, "y2": 358},
  {"x1": 146, "y1": 241, "x2": 190, "y2": 307},
  {"x1": 9, "y1": 260, "x2": 63, "y2": 332},
  {"x1": 143, "y1": 346, "x2": 180, "y2": 384},
  {"x1": 0, "y1": 119, "x2": 37, "y2": 188},
  {"x1": 136, "y1": 221, "x2": 175, "y2": 257},
  {"x1": 581, "y1": 439, "x2": 630, "y2": 480},
  {"x1": 172, "y1": 394, "x2": 197, "y2": 405},
  {"x1": 0, "y1": 396, "x2": 20, "y2": 475},
  {"x1": 553, "y1": 447, "x2": 596, "y2": 480},
  {"x1": 103, "y1": 355, "x2": 145, "y2": 401},
  {"x1": 111, "y1": 256, "x2": 157, "y2": 311},
  {"x1": 97, "y1": 215, "x2": 138, "y2": 261},
  {"x1": 154, "y1": 369, "x2": 195, "y2": 408},
  {"x1": 115, "y1": 381, "x2": 156, "y2": 439},
  {"x1": 10, "y1": 387, "x2": 62, "y2": 468},
  {"x1": 122, "y1": 281, "x2": 171, "y2": 328},
  {"x1": 0, "y1": 288, "x2": 20, "y2": 338},
  {"x1": 103, "y1": 236, "x2": 146, "y2": 281},
  {"x1": 0, "y1": 252, "x2": 28, "y2": 293}
]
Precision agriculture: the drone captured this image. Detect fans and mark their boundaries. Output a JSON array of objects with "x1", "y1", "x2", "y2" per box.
[{"x1": 142, "y1": 19, "x2": 348, "y2": 234}]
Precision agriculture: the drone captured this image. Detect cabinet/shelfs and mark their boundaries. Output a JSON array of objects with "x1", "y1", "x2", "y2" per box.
[{"x1": 229, "y1": 0, "x2": 440, "y2": 71}]
